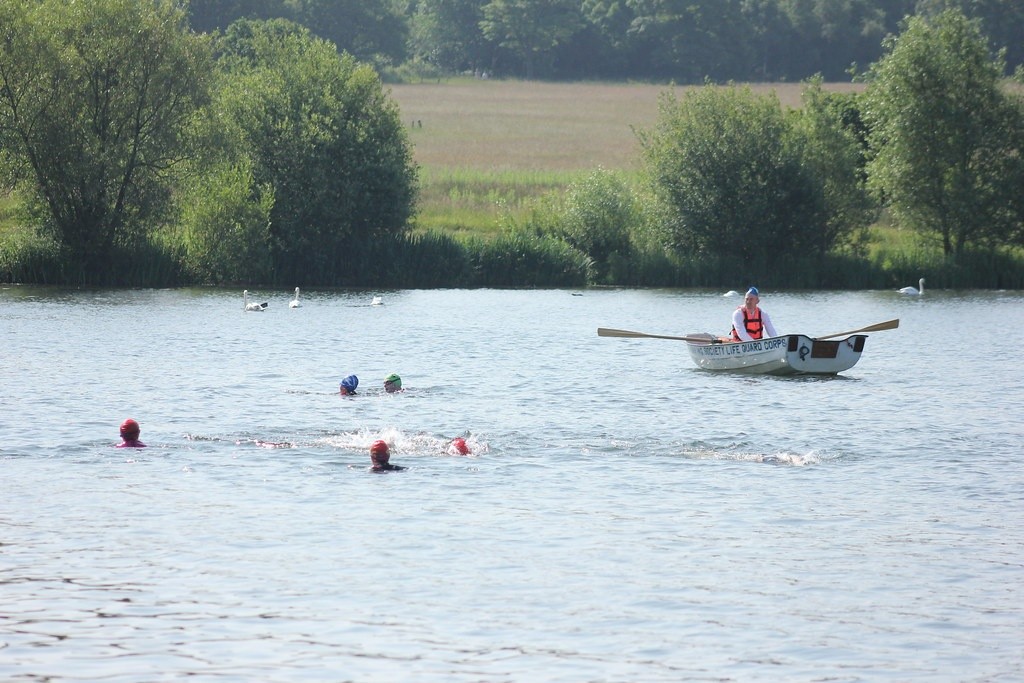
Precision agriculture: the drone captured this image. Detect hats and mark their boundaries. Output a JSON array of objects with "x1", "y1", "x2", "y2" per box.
[
  {"x1": 452, "y1": 438, "x2": 469, "y2": 455},
  {"x1": 384, "y1": 374, "x2": 401, "y2": 386},
  {"x1": 370, "y1": 440, "x2": 390, "y2": 463},
  {"x1": 748, "y1": 286, "x2": 759, "y2": 296},
  {"x1": 340, "y1": 375, "x2": 359, "y2": 391}
]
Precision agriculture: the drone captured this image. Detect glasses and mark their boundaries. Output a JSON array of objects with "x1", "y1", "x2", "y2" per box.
[{"x1": 384, "y1": 381, "x2": 392, "y2": 385}]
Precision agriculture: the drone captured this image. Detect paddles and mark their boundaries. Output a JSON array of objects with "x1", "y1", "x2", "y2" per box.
[
  {"x1": 815, "y1": 318, "x2": 900, "y2": 340},
  {"x1": 597, "y1": 327, "x2": 723, "y2": 344}
]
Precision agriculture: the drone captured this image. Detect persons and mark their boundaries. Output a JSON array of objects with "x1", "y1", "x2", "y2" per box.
[
  {"x1": 729, "y1": 287, "x2": 777, "y2": 343},
  {"x1": 383, "y1": 374, "x2": 405, "y2": 393},
  {"x1": 370, "y1": 440, "x2": 407, "y2": 472},
  {"x1": 116, "y1": 420, "x2": 146, "y2": 447},
  {"x1": 451, "y1": 436, "x2": 469, "y2": 455},
  {"x1": 339, "y1": 375, "x2": 359, "y2": 396}
]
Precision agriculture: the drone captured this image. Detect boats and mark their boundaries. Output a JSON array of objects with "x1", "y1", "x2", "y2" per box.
[{"x1": 683, "y1": 330, "x2": 869, "y2": 376}]
